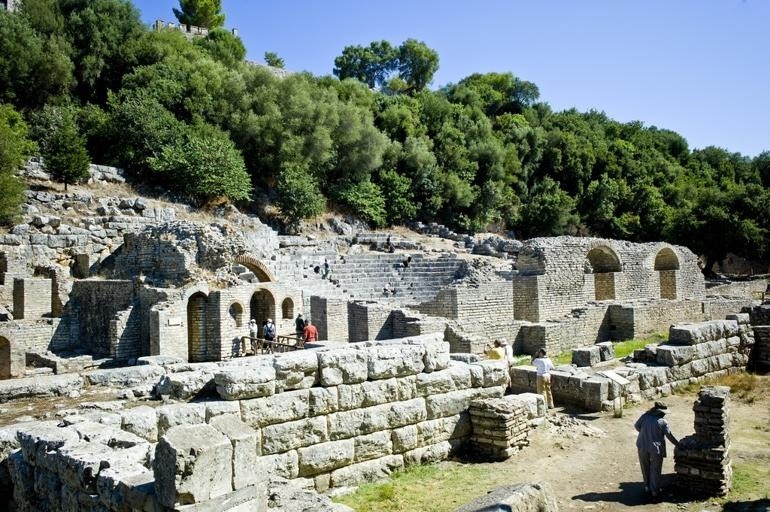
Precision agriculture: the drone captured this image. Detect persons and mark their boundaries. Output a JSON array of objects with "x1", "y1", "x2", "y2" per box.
[
  {"x1": 248, "y1": 318, "x2": 258, "y2": 354},
  {"x1": 261, "y1": 317, "x2": 276, "y2": 354},
  {"x1": 633, "y1": 400, "x2": 680, "y2": 500},
  {"x1": 483, "y1": 339, "x2": 506, "y2": 360},
  {"x1": 302, "y1": 319, "x2": 319, "y2": 345},
  {"x1": 293, "y1": 313, "x2": 305, "y2": 347},
  {"x1": 530, "y1": 347, "x2": 557, "y2": 410},
  {"x1": 321, "y1": 258, "x2": 332, "y2": 279}
]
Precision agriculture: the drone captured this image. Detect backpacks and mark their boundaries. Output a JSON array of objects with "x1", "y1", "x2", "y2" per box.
[{"x1": 265, "y1": 324, "x2": 274, "y2": 339}]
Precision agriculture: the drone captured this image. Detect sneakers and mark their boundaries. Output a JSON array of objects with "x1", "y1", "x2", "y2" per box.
[{"x1": 652, "y1": 488, "x2": 663, "y2": 497}]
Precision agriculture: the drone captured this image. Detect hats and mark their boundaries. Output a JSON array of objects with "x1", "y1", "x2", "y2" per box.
[
  {"x1": 494, "y1": 340, "x2": 501, "y2": 345},
  {"x1": 250, "y1": 319, "x2": 256, "y2": 321},
  {"x1": 501, "y1": 339, "x2": 508, "y2": 344},
  {"x1": 652, "y1": 402, "x2": 671, "y2": 415},
  {"x1": 267, "y1": 319, "x2": 272, "y2": 323}
]
